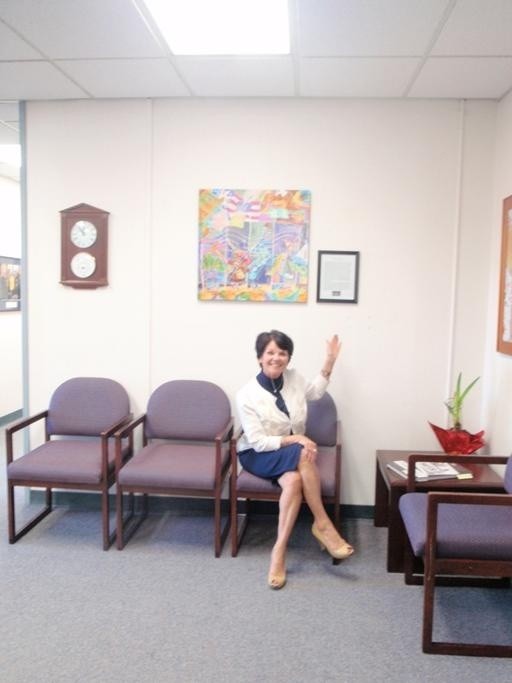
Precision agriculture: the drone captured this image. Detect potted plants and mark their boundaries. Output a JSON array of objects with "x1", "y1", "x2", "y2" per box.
[{"x1": 427, "y1": 370, "x2": 487, "y2": 457}]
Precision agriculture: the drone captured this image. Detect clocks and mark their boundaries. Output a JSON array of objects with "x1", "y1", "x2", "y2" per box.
[{"x1": 59, "y1": 201, "x2": 109, "y2": 288}]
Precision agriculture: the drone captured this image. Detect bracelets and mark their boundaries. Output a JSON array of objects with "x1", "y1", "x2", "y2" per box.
[{"x1": 319, "y1": 368, "x2": 331, "y2": 377}]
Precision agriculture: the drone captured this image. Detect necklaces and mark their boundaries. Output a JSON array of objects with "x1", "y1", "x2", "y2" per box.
[{"x1": 270, "y1": 378, "x2": 283, "y2": 393}]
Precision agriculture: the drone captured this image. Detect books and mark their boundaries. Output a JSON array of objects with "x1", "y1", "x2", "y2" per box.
[{"x1": 386, "y1": 457, "x2": 474, "y2": 483}]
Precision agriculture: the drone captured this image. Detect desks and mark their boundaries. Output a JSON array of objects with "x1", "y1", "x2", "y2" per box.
[{"x1": 372, "y1": 447, "x2": 507, "y2": 572}]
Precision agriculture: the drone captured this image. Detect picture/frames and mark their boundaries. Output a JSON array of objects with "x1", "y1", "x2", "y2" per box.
[
  {"x1": 315, "y1": 248, "x2": 359, "y2": 303},
  {"x1": 0, "y1": 255, "x2": 21, "y2": 310}
]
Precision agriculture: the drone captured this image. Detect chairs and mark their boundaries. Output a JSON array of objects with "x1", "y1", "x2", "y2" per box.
[{"x1": 397, "y1": 453, "x2": 512, "y2": 659}]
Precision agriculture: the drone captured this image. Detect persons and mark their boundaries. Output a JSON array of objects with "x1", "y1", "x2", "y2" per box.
[{"x1": 236, "y1": 325, "x2": 356, "y2": 592}]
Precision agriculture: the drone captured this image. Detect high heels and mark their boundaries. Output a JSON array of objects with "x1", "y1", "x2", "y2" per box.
[
  {"x1": 268, "y1": 551, "x2": 287, "y2": 589},
  {"x1": 311, "y1": 524, "x2": 354, "y2": 559}
]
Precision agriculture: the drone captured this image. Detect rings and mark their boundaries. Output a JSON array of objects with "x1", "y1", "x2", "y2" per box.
[{"x1": 312, "y1": 448, "x2": 319, "y2": 453}]
